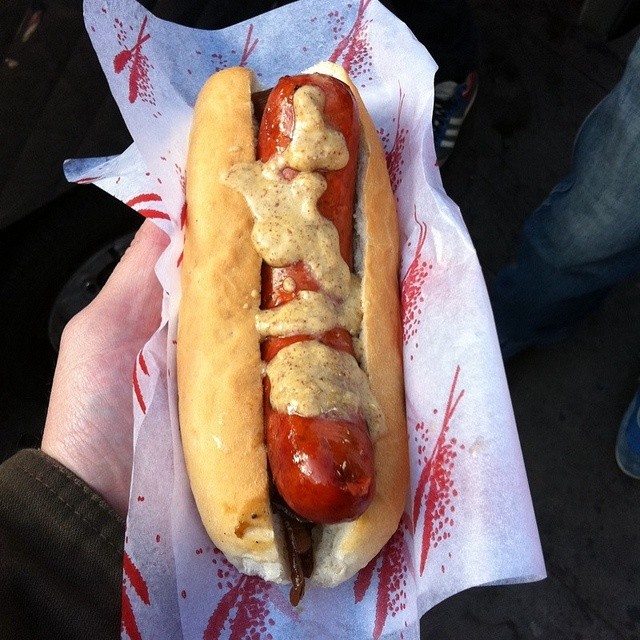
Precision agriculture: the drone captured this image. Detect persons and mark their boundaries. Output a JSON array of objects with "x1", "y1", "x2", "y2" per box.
[
  {"x1": 489, "y1": 35, "x2": 640, "y2": 482},
  {"x1": 0, "y1": 217, "x2": 171, "y2": 640}
]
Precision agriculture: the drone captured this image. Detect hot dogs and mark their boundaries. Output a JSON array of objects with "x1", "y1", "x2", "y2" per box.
[{"x1": 177, "y1": 60, "x2": 409, "y2": 585}]
